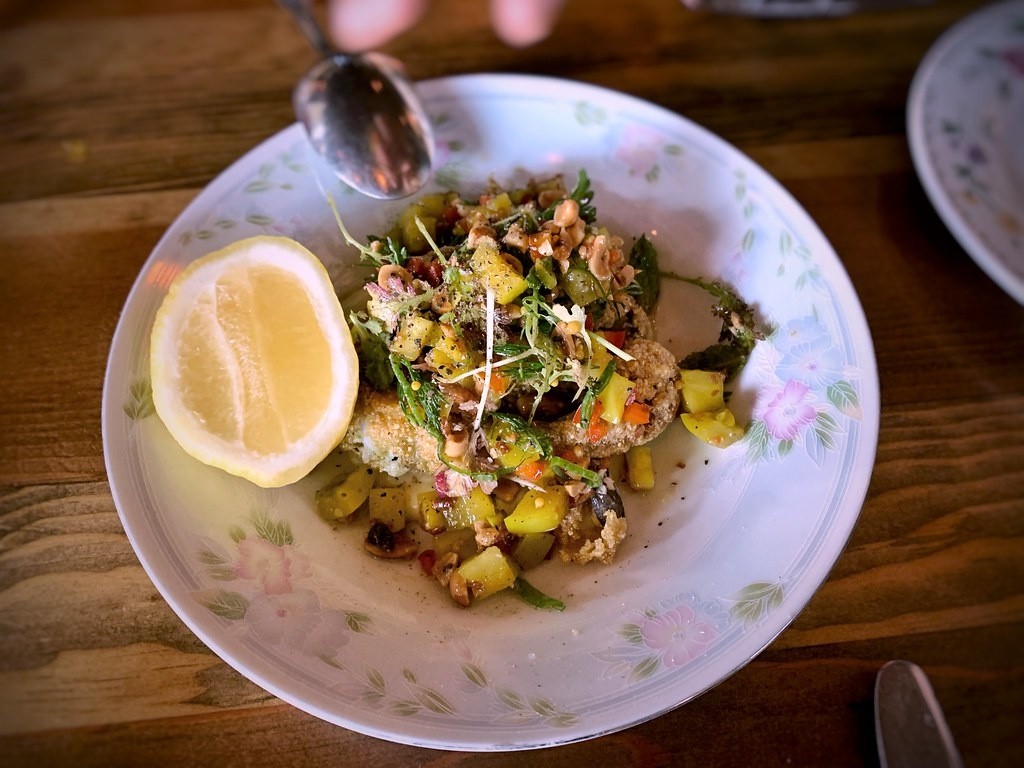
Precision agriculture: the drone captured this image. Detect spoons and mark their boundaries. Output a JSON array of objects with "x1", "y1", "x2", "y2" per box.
[{"x1": 282, "y1": 0, "x2": 436, "y2": 201}]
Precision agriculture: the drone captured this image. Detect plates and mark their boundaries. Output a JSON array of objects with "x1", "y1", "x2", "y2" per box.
[
  {"x1": 101, "y1": 72, "x2": 881, "y2": 753},
  {"x1": 906, "y1": 0, "x2": 1024, "y2": 308}
]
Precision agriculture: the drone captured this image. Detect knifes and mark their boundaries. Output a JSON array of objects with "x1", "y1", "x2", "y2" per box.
[{"x1": 874, "y1": 660, "x2": 963, "y2": 768}]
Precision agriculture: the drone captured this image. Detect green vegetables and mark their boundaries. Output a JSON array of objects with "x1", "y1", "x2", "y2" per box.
[{"x1": 328, "y1": 170, "x2": 762, "y2": 485}]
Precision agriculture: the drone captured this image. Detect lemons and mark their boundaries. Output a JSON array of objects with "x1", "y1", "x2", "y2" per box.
[{"x1": 149, "y1": 235, "x2": 358, "y2": 489}]
